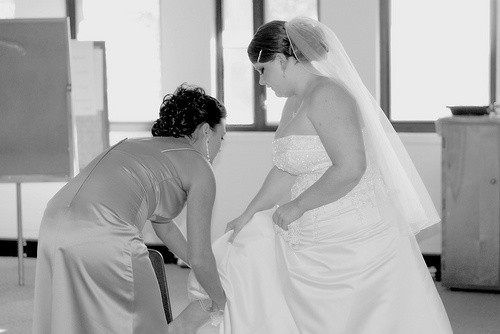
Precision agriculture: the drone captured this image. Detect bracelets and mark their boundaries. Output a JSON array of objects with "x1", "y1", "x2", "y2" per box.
[{"x1": 211, "y1": 290, "x2": 226, "y2": 305}]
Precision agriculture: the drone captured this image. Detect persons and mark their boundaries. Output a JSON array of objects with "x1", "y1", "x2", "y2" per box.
[
  {"x1": 32, "y1": 81, "x2": 226, "y2": 334},
  {"x1": 224, "y1": 15, "x2": 458, "y2": 334}
]
